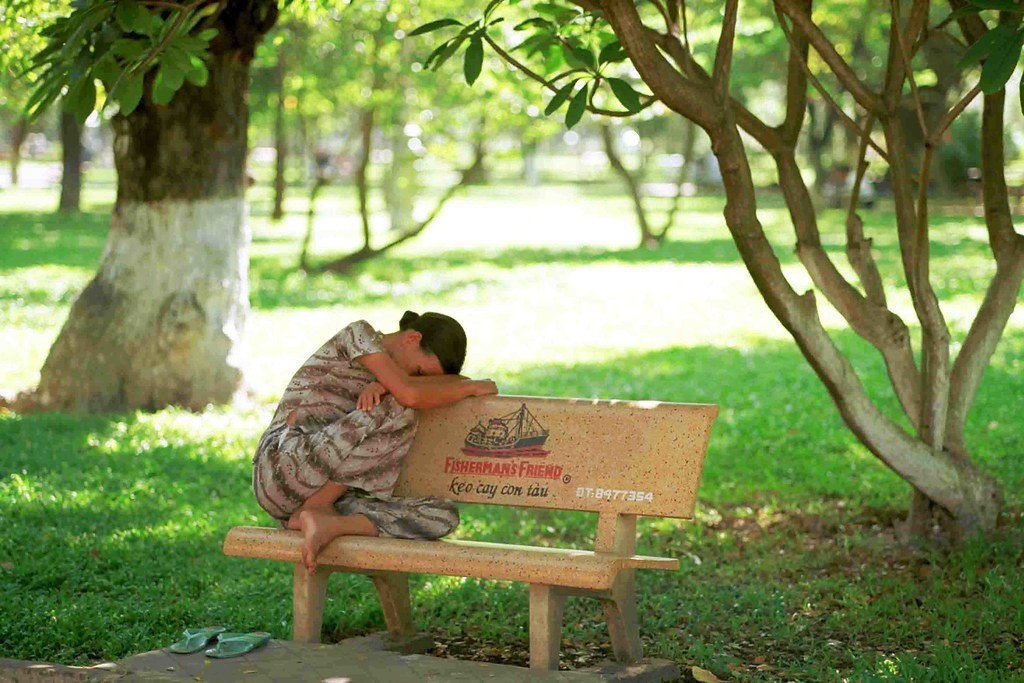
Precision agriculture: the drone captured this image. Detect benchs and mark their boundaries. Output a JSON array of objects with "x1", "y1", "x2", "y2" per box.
[{"x1": 222, "y1": 391, "x2": 720, "y2": 670}]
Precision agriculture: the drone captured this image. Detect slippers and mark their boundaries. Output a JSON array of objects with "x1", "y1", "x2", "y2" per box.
[
  {"x1": 205, "y1": 630, "x2": 272, "y2": 658},
  {"x1": 169, "y1": 626, "x2": 226, "y2": 654}
]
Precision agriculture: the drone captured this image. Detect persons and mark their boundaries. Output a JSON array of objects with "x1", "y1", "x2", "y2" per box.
[
  {"x1": 252, "y1": 311, "x2": 498, "y2": 574},
  {"x1": 835, "y1": 165, "x2": 873, "y2": 208}
]
